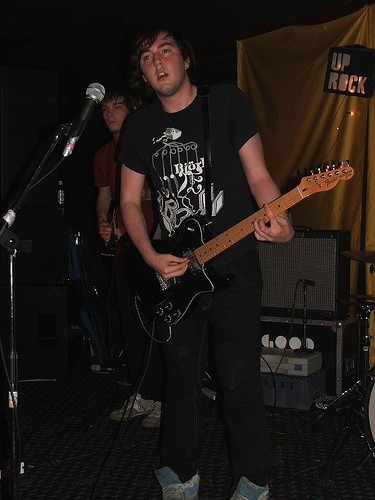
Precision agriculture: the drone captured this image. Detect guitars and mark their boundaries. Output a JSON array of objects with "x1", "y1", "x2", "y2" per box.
[
  {"x1": 106, "y1": 184, "x2": 151, "y2": 248},
  {"x1": 123, "y1": 160, "x2": 355, "y2": 325}
]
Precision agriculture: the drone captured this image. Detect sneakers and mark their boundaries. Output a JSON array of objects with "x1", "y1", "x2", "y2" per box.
[
  {"x1": 110, "y1": 393, "x2": 154, "y2": 421},
  {"x1": 141, "y1": 401, "x2": 162, "y2": 428},
  {"x1": 163, "y1": 467, "x2": 200, "y2": 500},
  {"x1": 230, "y1": 475, "x2": 269, "y2": 500}
]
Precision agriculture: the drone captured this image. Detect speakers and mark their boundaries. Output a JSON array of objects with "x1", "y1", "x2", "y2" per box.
[
  {"x1": 1, "y1": 66, "x2": 61, "y2": 207},
  {"x1": 0, "y1": 284, "x2": 73, "y2": 378},
  {"x1": 0, "y1": 208, "x2": 65, "y2": 285},
  {"x1": 258, "y1": 230, "x2": 350, "y2": 319}
]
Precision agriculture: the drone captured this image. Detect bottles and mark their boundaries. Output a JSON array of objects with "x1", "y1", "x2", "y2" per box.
[{"x1": 56, "y1": 174, "x2": 65, "y2": 207}]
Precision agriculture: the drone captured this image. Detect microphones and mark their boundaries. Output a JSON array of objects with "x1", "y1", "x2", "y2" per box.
[
  {"x1": 63, "y1": 82, "x2": 105, "y2": 159},
  {"x1": 299, "y1": 277, "x2": 316, "y2": 287}
]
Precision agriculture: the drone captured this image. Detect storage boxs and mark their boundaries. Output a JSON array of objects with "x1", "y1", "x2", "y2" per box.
[{"x1": 259, "y1": 315, "x2": 361, "y2": 397}]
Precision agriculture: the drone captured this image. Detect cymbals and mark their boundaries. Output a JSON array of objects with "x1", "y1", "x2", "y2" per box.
[
  {"x1": 340, "y1": 250, "x2": 375, "y2": 263},
  {"x1": 336, "y1": 294, "x2": 375, "y2": 309}
]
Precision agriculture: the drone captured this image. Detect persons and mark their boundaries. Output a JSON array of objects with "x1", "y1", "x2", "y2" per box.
[
  {"x1": 93, "y1": 86, "x2": 165, "y2": 427},
  {"x1": 118, "y1": 22, "x2": 295, "y2": 500}
]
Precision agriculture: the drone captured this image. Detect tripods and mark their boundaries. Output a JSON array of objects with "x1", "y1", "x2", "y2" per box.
[{"x1": 319, "y1": 306, "x2": 375, "y2": 416}]
